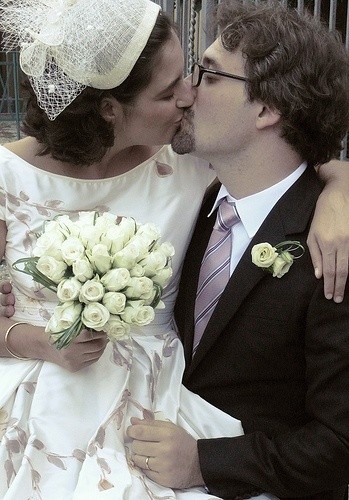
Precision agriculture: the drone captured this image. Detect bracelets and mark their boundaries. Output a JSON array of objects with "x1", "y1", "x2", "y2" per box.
[
  {"x1": 4, "y1": 322, "x2": 35, "y2": 361},
  {"x1": 0, "y1": 0, "x2": 349, "y2": 500}
]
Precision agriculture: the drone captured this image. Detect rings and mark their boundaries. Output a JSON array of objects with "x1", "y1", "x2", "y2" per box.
[{"x1": 145, "y1": 457, "x2": 150, "y2": 471}]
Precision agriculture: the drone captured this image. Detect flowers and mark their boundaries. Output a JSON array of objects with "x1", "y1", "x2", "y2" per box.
[
  {"x1": 12, "y1": 211, "x2": 175, "y2": 351},
  {"x1": 251, "y1": 241, "x2": 305, "y2": 278}
]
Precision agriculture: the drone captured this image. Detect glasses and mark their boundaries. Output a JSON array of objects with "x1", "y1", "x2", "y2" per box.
[{"x1": 191, "y1": 63, "x2": 250, "y2": 87}]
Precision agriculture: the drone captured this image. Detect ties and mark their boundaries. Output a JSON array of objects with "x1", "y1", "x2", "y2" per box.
[{"x1": 192, "y1": 198, "x2": 241, "y2": 359}]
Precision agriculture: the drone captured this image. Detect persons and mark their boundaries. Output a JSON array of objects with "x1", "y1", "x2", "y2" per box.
[{"x1": 126, "y1": 0, "x2": 349, "y2": 500}]
